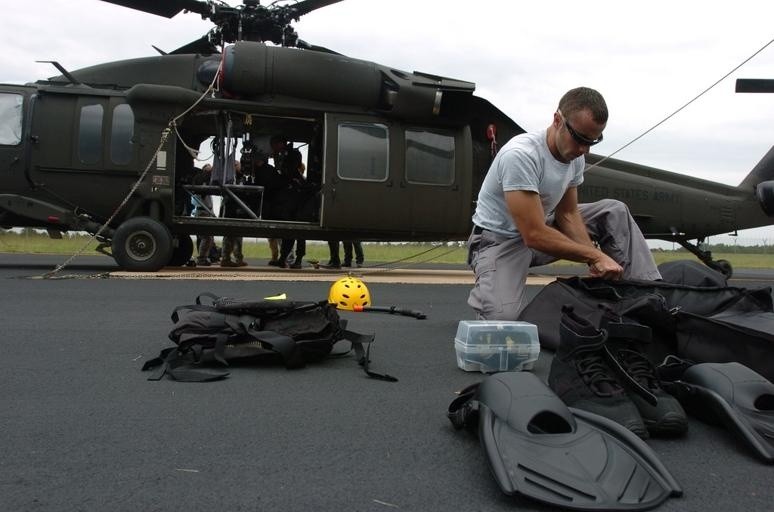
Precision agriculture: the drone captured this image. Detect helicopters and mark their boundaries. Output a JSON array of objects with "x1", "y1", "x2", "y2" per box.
[{"x1": 2, "y1": 0, "x2": 774, "y2": 272}]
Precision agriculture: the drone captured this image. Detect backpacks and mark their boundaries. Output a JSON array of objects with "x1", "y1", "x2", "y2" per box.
[{"x1": 167, "y1": 292, "x2": 348, "y2": 367}]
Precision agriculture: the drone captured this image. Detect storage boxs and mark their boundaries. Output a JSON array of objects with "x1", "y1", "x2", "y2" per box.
[{"x1": 454, "y1": 320, "x2": 541, "y2": 371}]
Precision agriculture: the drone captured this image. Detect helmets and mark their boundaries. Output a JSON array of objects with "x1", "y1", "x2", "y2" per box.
[{"x1": 327, "y1": 276, "x2": 372, "y2": 312}]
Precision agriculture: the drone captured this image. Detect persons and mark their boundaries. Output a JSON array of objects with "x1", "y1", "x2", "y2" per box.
[
  {"x1": 463, "y1": 86, "x2": 665, "y2": 322},
  {"x1": 193, "y1": 133, "x2": 364, "y2": 268}
]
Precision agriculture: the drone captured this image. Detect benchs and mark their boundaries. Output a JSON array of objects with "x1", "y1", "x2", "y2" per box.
[{"x1": 179, "y1": 123, "x2": 266, "y2": 220}]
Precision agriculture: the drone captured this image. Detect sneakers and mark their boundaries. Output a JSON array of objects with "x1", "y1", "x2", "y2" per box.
[
  {"x1": 547, "y1": 302, "x2": 690, "y2": 442},
  {"x1": 197, "y1": 255, "x2": 364, "y2": 269}
]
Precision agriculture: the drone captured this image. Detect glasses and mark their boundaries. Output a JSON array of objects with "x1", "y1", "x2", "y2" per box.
[{"x1": 557, "y1": 108, "x2": 603, "y2": 146}]
